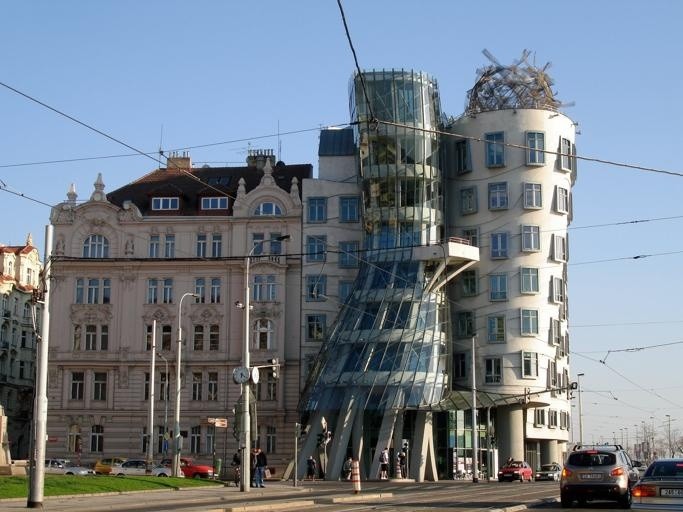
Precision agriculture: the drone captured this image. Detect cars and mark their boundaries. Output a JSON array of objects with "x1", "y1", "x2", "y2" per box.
[
  {"x1": 497, "y1": 461, "x2": 532, "y2": 483},
  {"x1": 631, "y1": 458, "x2": 683, "y2": 511},
  {"x1": 93, "y1": 456, "x2": 131, "y2": 475},
  {"x1": 44, "y1": 458, "x2": 95, "y2": 476},
  {"x1": 635, "y1": 462, "x2": 647, "y2": 472},
  {"x1": 108, "y1": 458, "x2": 184, "y2": 478},
  {"x1": 535, "y1": 463, "x2": 562, "y2": 482}
]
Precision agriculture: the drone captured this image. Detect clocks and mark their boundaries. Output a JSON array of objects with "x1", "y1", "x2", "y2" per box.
[{"x1": 232, "y1": 365, "x2": 249, "y2": 383}]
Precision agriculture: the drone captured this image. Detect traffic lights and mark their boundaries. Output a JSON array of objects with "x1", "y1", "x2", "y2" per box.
[
  {"x1": 490, "y1": 436, "x2": 496, "y2": 445},
  {"x1": 297, "y1": 424, "x2": 311, "y2": 436},
  {"x1": 267, "y1": 357, "x2": 277, "y2": 379}
]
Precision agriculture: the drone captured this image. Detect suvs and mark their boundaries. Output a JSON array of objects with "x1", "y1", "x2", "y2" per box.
[
  {"x1": 158, "y1": 458, "x2": 214, "y2": 480},
  {"x1": 560, "y1": 444, "x2": 642, "y2": 509}
]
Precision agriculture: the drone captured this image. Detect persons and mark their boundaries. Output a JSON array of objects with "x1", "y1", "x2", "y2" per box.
[
  {"x1": 401, "y1": 443, "x2": 408, "y2": 476},
  {"x1": 232, "y1": 446, "x2": 267, "y2": 487},
  {"x1": 343, "y1": 457, "x2": 353, "y2": 481},
  {"x1": 398, "y1": 449, "x2": 406, "y2": 479},
  {"x1": 307, "y1": 455, "x2": 316, "y2": 481},
  {"x1": 502, "y1": 457, "x2": 512, "y2": 469},
  {"x1": 379, "y1": 447, "x2": 389, "y2": 480}
]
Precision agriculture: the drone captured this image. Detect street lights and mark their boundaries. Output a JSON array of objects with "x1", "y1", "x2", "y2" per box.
[
  {"x1": 599, "y1": 416, "x2": 655, "y2": 462},
  {"x1": 171, "y1": 292, "x2": 201, "y2": 478},
  {"x1": 570, "y1": 404, "x2": 575, "y2": 450},
  {"x1": 232, "y1": 233, "x2": 293, "y2": 493},
  {"x1": 577, "y1": 373, "x2": 584, "y2": 450},
  {"x1": 156, "y1": 351, "x2": 168, "y2": 461},
  {"x1": 470, "y1": 331, "x2": 480, "y2": 483},
  {"x1": 665, "y1": 414, "x2": 670, "y2": 458}
]
